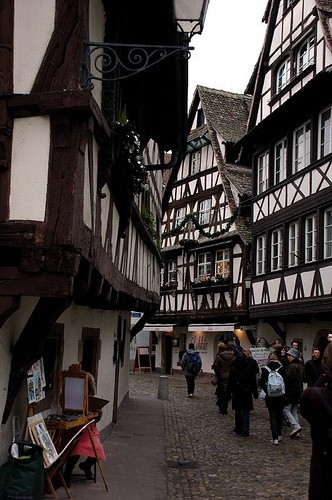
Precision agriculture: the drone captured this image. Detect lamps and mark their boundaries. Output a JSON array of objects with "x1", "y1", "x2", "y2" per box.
[{"x1": 80, "y1": 0, "x2": 210, "y2": 90}]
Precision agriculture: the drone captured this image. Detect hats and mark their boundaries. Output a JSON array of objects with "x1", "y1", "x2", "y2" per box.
[{"x1": 286, "y1": 348, "x2": 300, "y2": 358}]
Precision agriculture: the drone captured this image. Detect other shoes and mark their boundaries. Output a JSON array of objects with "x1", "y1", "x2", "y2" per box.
[
  {"x1": 187, "y1": 392, "x2": 194, "y2": 397},
  {"x1": 290, "y1": 425, "x2": 302, "y2": 438},
  {"x1": 271, "y1": 435, "x2": 283, "y2": 445},
  {"x1": 217, "y1": 409, "x2": 229, "y2": 414},
  {"x1": 233, "y1": 428, "x2": 249, "y2": 437}
]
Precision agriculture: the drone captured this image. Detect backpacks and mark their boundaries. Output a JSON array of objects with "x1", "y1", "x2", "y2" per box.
[
  {"x1": 262, "y1": 365, "x2": 286, "y2": 396},
  {"x1": 186, "y1": 352, "x2": 201, "y2": 375}
]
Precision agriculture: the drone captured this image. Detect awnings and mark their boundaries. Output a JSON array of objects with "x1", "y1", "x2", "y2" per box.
[
  {"x1": 143, "y1": 323, "x2": 178, "y2": 332},
  {"x1": 188, "y1": 323, "x2": 256, "y2": 332}
]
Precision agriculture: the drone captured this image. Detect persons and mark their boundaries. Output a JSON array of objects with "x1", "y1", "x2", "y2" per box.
[
  {"x1": 59, "y1": 362, "x2": 99, "y2": 489},
  {"x1": 212, "y1": 332, "x2": 332, "y2": 500},
  {"x1": 180, "y1": 342, "x2": 202, "y2": 398}
]
